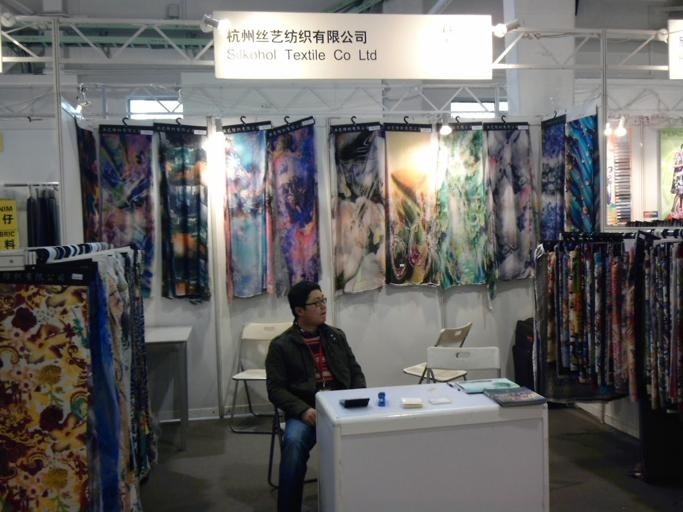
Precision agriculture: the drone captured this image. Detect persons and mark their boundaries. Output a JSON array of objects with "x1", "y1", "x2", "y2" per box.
[{"x1": 261, "y1": 278, "x2": 369, "y2": 512}]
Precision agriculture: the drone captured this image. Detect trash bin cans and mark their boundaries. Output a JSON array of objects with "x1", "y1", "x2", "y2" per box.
[{"x1": 511, "y1": 318, "x2": 566, "y2": 409}]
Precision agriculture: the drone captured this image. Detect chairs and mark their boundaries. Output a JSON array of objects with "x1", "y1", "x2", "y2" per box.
[
  {"x1": 265, "y1": 403, "x2": 317, "y2": 491},
  {"x1": 424, "y1": 341, "x2": 505, "y2": 384},
  {"x1": 228, "y1": 321, "x2": 297, "y2": 437},
  {"x1": 397, "y1": 320, "x2": 474, "y2": 385}
]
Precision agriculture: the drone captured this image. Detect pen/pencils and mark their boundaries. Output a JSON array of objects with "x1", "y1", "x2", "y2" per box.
[{"x1": 446, "y1": 382, "x2": 460, "y2": 391}]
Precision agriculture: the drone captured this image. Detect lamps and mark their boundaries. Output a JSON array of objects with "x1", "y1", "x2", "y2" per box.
[
  {"x1": 596, "y1": 115, "x2": 631, "y2": 139},
  {"x1": 436, "y1": 109, "x2": 457, "y2": 138},
  {"x1": 487, "y1": 16, "x2": 526, "y2": 38},
  {"x1": 199, "y1": 17, "x2": 233, "y2": 37},
  {"x1": 71, "y1": 83, "x2": 95, "y2": 117}
]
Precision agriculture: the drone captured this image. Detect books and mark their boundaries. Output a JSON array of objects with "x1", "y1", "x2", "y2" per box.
[
  {"x1": 482, "y1": 385, "x2": 547, "y2": 410},
  {"x1": 453, "y1": 377, "x2": 525, "y2": 395}
]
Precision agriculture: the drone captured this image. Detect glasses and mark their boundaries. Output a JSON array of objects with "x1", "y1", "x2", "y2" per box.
[{"x1": 297, "y1": 298, "x2": 329, "y2": 307}]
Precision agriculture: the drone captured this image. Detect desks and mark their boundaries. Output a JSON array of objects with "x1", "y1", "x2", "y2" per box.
[
  {"x1": 139, "y1": 326, "x2": 196, "y2": 451},
  {"x1": 312, "y1": 374, "x2": 551, "y2": 511}
]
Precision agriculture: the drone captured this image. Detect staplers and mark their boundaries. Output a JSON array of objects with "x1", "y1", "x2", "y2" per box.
[{"x1": 378, "y1": 392, "x2": 385, "y2": 407}]
